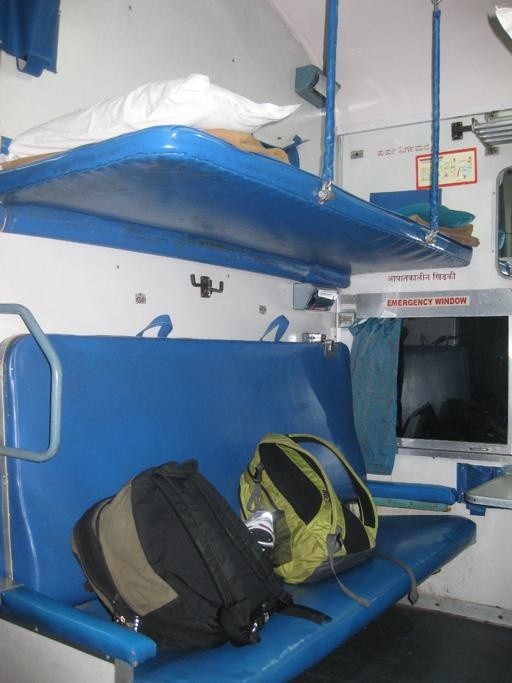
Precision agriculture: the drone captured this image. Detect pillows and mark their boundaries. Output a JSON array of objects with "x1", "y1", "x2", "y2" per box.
[{"x1": 3, "y1": 73, "x2": 301, "y2": 161}]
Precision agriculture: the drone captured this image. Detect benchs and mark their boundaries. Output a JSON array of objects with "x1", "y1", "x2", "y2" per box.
[{"x1": 1, "y1": 334, "x2": 478, "y2": 682}]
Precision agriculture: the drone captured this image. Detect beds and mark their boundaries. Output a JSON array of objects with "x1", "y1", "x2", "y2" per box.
[{"x1": 0, "y1": 133, "x2": 475, "y2": 290}]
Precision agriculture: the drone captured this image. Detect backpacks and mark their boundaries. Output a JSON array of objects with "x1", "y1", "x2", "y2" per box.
[
  {"x1": 71, "y1": 458, "x2": 333, "y2": 655},
  {"x1": 239, "y1": 431, "x2": 421, "y2": 608}
]
